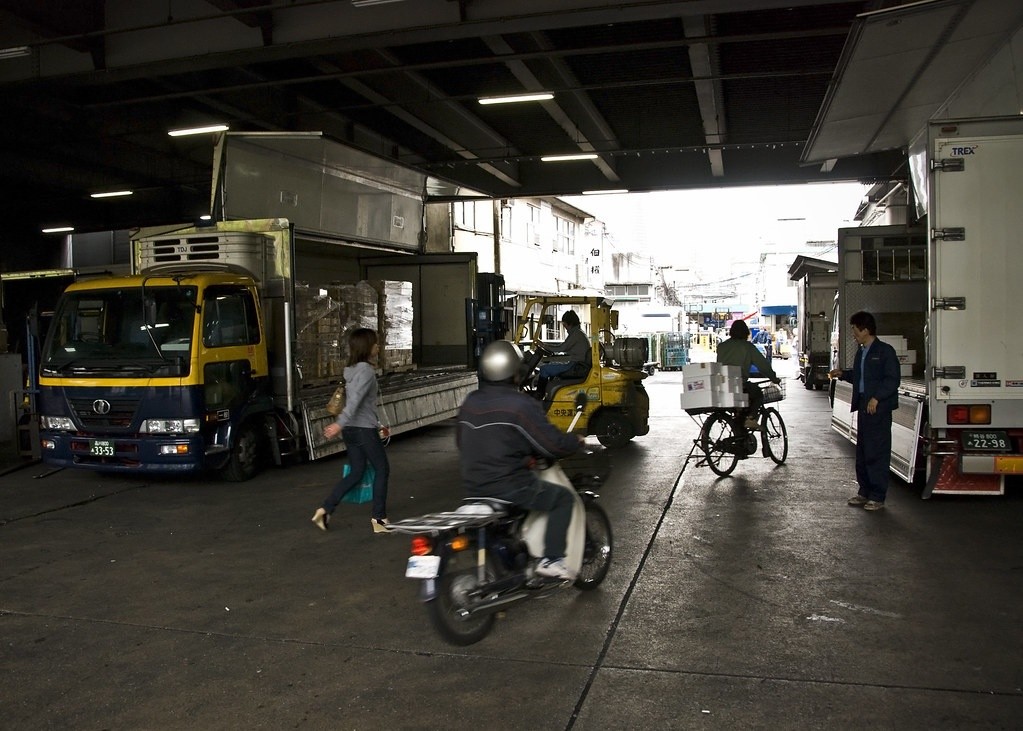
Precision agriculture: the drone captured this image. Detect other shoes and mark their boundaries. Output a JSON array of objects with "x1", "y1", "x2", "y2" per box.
[{"x1": 525, "y1": 390, "x2": 543, "y2": 400}]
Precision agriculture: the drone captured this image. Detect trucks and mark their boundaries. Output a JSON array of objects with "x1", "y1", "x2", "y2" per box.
[
  {"x1": 796, "y1": 0, "x2": 1023, "y2": 503},
  {"x1": 31, "y1": 128, "x2": 479, "y2": 483},
  {"x1": 798, "y1": 270, "x2": 840, "y2": 391}
]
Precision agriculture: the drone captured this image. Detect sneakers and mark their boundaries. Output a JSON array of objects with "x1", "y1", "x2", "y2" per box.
[
  {"x1": 534, "y1": 556, "x2": 578, "y2": 581},
  {"x1": 743, "y1": 416, "x2": 759, "y2": 429},
  {"x1": 864, "y1": 500, "x2": 888, "y2": 511},
  {"x1": 847, "y1": 494, "x2": 868, "y2": 505}
]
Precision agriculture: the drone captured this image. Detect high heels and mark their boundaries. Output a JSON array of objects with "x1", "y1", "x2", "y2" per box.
[
  {"x1": 311, "y1": 507, "x2": 330, "y2": 531},
  {"x1": 370, "y1": 518, "x2": 396, "y2": 534}
]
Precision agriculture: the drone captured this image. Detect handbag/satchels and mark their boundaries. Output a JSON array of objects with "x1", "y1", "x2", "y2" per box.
[{"x1": 326, "y1": 377, "x2": 346, "y2": 417}]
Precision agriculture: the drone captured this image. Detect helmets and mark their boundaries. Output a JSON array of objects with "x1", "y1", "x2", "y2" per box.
[{"x1": 477, "y1": 340, "x2": 524, "y2": 381}]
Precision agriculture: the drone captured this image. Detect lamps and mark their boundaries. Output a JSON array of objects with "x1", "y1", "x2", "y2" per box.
[
  {"x1": 0, "y1": 45, "x2": 32, "y2": 60},
  {"x1": 167, "y1": 122, "x2": 231, "y2": 137},
  {"x1": 477, "y1": 90, "x2": 555, "y2": 105},
  {"x1": 540, "y1": 152, "x2": 601, "y2": 161}
]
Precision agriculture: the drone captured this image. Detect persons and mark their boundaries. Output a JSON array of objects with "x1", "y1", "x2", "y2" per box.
[
  {"x1": 524, "y1": 310, "x2": 591, "y2": 399},
  {"x1": 830, "y1": 311, "x2": 901, "y2": 512},
  {"x1": 311, "y1": 328, "x2": 400, "y2": 533},
  {"x1": 717, "y1": 319, "x2": 781, "y2": 434},
  {"x1": 456, "y1": 340, "x2": 602, "y2": 590}
]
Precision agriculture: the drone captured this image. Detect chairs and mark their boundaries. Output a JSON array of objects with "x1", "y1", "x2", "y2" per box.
[
  {"x1": 120, "y1": 300, "x2": 143, "y2": 344},
  {"x1": 206, "y1": 300, "x2": 245, "y2": 345}
]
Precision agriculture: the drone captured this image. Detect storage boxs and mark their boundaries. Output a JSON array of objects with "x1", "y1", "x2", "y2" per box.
[
  {"x1": 811, "y1": 319, "x2": 830, "y2": 352},
  {"x1": 680, "y1": 361, "x2": 749, "y2": 410},
  {"x1": 296, "y1": 279, "x2": 414, "y2": 378},
  {"x1": 876, "y1": 335, "x2": 917, "y2": 377},
  {"x1": 665, "y1": 358, "x2": 686, "y2": 365},
  {"x1": 664, "y1": 347, "x2": 687, "y2": 359}
]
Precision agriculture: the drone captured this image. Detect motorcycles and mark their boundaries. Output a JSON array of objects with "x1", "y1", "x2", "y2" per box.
[{"x1": 384, "y1": 391, "x2": 614, "y2": 645}]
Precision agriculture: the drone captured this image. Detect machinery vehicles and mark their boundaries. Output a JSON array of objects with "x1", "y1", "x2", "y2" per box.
[{"x1": 389, "y1": 271, "x2": 650, "y2": 448}]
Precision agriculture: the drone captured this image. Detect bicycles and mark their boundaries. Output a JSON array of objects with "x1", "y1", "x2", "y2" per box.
[{"x1": 693, "y1": 376, "x2": 789, "y2": 478}]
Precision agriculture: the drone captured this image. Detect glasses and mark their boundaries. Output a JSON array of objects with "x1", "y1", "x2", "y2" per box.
[{"x1": 563, "y1": 324, "x2": 568, "y2": 327}]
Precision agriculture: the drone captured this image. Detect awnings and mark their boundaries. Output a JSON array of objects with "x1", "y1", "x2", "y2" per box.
[{"x1": 760, "y1": 305, "x2": 798, "y2": 317}]
